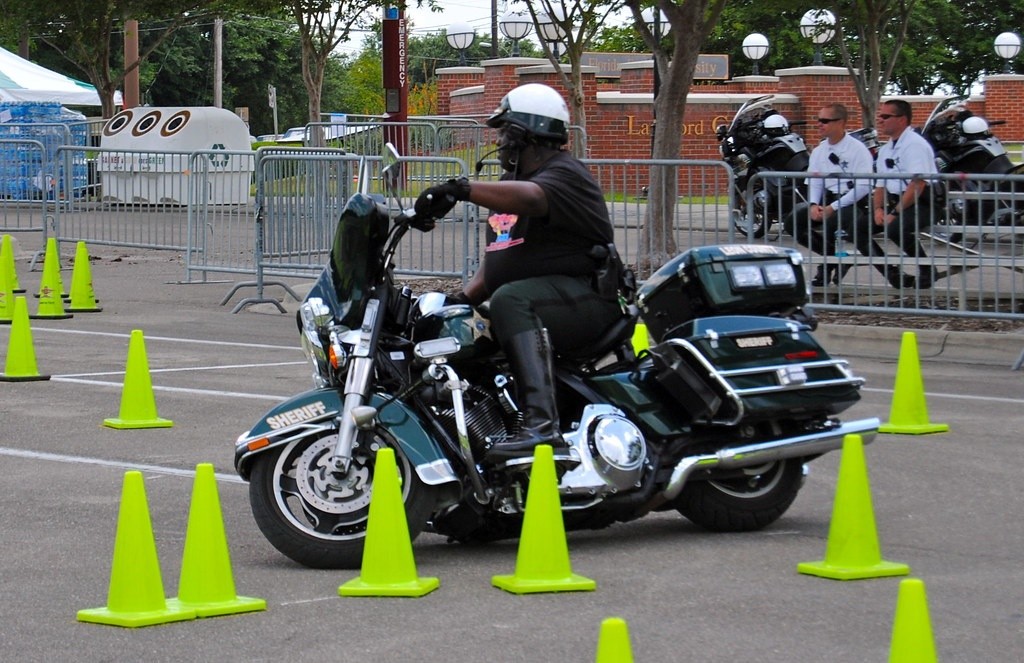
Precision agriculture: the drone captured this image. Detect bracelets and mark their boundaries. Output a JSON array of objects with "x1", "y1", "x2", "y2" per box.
[{"x1": 875, "y1": 206, "x2": 883, "y2": 210}]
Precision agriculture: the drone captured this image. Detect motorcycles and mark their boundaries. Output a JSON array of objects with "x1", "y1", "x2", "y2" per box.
[
  {"x1": 234, "y1": 143, "x2": 882, "y2": 570},
  {"x1": 914, "y1": 94, "x2": 1024, "y2": 243},
  {"x1": 714, "y1": 95, "x2": 881, "y2": 238}
]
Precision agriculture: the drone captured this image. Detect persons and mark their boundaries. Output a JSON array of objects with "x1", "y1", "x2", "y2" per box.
[
  {"x1": 846, "y1": 100, "x2": 946, "y2": 290},
  {"x1": 785, "y1": 103, "x2": 874, "y2": 287},
  {"x1": 414, "y1": 83, "x2": 626, "y2": 449}
]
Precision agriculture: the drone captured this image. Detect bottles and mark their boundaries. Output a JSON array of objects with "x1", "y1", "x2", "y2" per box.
[{"x1": 834, "y1": 228, "x2": 847, "y2": 257}]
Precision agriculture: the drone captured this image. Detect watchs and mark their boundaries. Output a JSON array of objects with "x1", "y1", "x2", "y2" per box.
[{"x1": 891, "y1": 208, "x2": 900, "y2": 217}]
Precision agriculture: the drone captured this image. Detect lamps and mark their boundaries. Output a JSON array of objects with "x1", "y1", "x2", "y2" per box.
[
  {"x1": 799, "y1": 9, "x2": 837, "y2": 65},
  {"x1": 538, "y1": 15, "x2": 568, "y2": 58},
  {"x1": 993, "y1": 30, "x2": 1021, "y2": 73},
  {"x1": 444, "y1": 22, "x2": 476, "y2": 66},
  {"x1": 499, "y1": 9, "x2": 534, "y2": 57},
  {"x1": 741, "y1": 33, "x2": 769, "y2": 75}
]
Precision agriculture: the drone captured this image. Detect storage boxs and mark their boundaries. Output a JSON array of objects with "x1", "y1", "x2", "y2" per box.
[
  {"x1": 657, "y1": 314, "x2": 861, "y2": 421},
  {"x1": 637, "y1": 241, "x2": 806, "y2": 332}
]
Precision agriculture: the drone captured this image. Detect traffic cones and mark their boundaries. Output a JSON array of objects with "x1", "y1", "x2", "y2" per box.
[
  {"x1": 338, "y1": 447, "x2": 442, "y2": 598},
  {"x1": 888, "y1": 576, "x2": 939, "y2": 663},
  {"x1": 103, "y1": 329, "x2": 174, "y2": 429},
  {"x1": 0, "y1": 296, "x2": 54, "y2": 382},
  {"x1": 166, "y1": 462, "x2": 266, "y2": 618},
  {"x1": 76, "y1": 472, "x2": 197, "y2": 629},
  {"x1": 488, "y1": 445, "x2": 597, "y2": 595},
  {"x1": 796, "y1": 434, "x2": 908, "y2": 580},
  {"x1": 594, "y1": 618, "x2": 633, "y2": 663},
  {"x1": 880, "y1": 332, "x2": 950, "y2": 436},
  {"x1": 29, "y1": 237, "x2": 102, "y2": 319},
  {"x1": 0, "y1": 233, "x2": 26, "y2": 324}
]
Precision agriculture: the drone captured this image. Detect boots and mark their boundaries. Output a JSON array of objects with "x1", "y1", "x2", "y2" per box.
[
  {"x1": 800, "y1": 229, "x2": 854, "y2": 285},
  {"x1": 857, "y1": 236, "x2": 916, "y2": 289},
  {"x1": 489, "y1": 326, "x2": 572, "y2": 460},
  {"x1": 907, "y1": 234, "x2": 937, "y2": 288}
]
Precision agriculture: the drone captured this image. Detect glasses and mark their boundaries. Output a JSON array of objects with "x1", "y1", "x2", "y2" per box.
[
  {"x1": 818, "y1": 117, "x2": 840, "y2": 124},
  {"x1": 879, "y1": 114, "x2": 903, "y2": 120}
]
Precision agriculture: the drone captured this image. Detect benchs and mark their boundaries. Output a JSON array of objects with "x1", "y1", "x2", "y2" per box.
[{"x1": 804, "y1": 190, "x2": 1024, "y2": 299}]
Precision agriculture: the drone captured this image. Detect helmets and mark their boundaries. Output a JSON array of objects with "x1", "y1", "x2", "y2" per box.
[
  {"x1": 486, "y1": 82, "x2": 571, "y2": 145},
  {"x1": 962, "y1": 116, "x2": 990, "y2": 140},
  {"x1": 764, "y1": 114, "x2": 791, "y2": 136}
]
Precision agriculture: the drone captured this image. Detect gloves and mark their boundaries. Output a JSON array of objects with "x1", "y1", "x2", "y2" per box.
[{"x1": 415, "y1": 177, "x2": 472, "y2": 219}]
